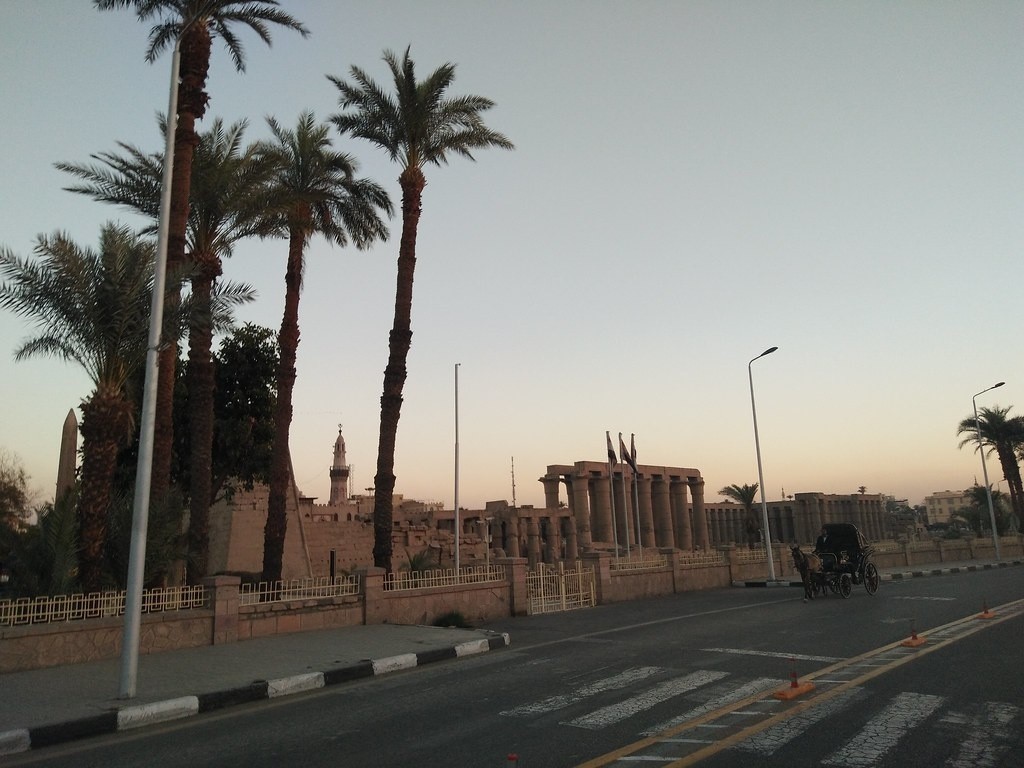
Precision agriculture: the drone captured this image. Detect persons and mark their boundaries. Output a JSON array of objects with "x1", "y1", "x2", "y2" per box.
[{"x1": 816, "y1": 527, "x2": 832, "y2": 553}]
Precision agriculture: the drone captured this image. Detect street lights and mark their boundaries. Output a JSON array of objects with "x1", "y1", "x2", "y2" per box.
[
  {"x1": 745, "y1": 347, "x2": 784, "y2": 585},
  {"x1": 973, "y1": 380, "x2": 1007, "y2": 572}
]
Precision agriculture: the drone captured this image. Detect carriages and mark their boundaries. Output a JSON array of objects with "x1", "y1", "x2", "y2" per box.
[{"x1": 789, "y1": 520, "x2": 880, "y2": 605}]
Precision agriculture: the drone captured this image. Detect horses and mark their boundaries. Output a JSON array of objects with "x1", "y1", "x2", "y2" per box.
[{"x1": 789, "y1": 545, "x2": 822, "y2": 603}]
